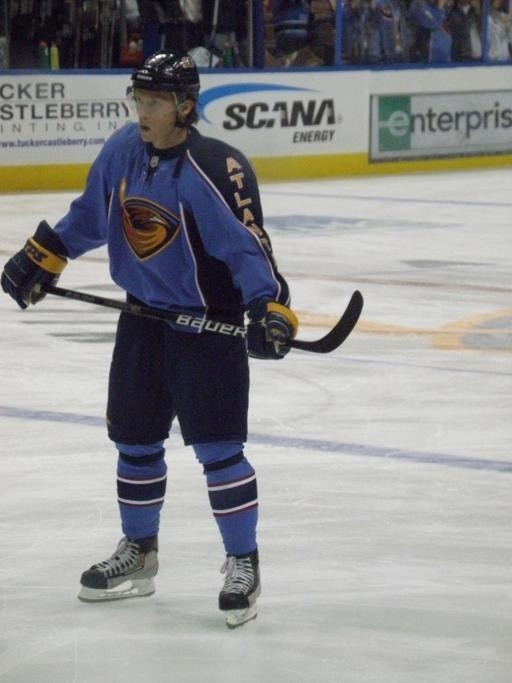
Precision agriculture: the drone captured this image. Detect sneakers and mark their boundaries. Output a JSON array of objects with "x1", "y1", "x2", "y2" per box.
[
  {"x1": 218, "y1": 549, "x2": 262, "y2": 612},
  {"x1": 79, "y1": 534, "x2": 160, "y2": 589}
]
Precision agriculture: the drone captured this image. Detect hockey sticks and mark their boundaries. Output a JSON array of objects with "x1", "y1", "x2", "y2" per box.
[{"x1": 44, "y1": 286, "x2": 362, "y2": 352}]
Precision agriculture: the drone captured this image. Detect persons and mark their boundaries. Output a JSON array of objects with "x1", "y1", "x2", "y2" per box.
[
  {"x1": 0, "y1": 0, "x2": 512, "y2": 68},
  {"x1": 0, "y1": 47, "x2": 302, "y2": 613}
]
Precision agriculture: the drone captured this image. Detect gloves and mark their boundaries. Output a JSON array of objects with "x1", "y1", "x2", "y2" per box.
[
  {"x1": 246, "y1": 298, "x2": 301, "y2": 360},
  {"x1": 0, "y1": 219, "x2": 69, "y2": 310}
]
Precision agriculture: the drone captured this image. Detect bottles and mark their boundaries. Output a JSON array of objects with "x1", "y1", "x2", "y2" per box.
[
  {"x1": 222, "y1": 33, "x2": 246, "y2": 69},
  {"x1": 39, "y1": 40, "x2": 50, "y2": 67},
  {"x1": 49, "y1": 42, "x2": 59, "y2": 69}
]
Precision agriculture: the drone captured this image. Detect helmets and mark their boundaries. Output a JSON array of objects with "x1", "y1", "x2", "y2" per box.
[{"x1": 129, "y1": 47, "x2": 201, "y2": 93}]
